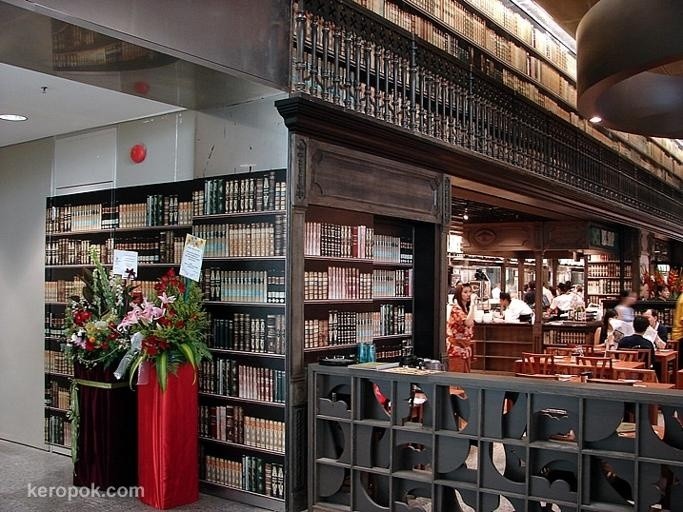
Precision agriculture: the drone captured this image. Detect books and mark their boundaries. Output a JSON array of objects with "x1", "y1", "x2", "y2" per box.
[{"x1": 541, "y1": 251, "x2": 682, "y2": 361}]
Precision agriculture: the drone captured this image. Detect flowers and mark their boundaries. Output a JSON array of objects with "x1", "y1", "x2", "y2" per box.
[
  {"x1": 124, "y1": 266, "x2": 213, "y2": 389},
  {"x1": 60, "y1": 246, "x2": 149, "y2": 365}
]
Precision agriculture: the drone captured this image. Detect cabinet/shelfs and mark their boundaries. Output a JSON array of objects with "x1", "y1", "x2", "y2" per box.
[
  {"x1": 468, "y1": 259, "x2": 682, "y2": 345},
  {"x1": 44, "y1": 168, "x2": 289, "y2": 509},
  {"x1": 294, "y1": 203, "x2": 415, "y2": 373}
]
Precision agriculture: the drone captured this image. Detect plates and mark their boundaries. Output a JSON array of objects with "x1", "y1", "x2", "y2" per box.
[
  {"x1": 611, "y1": 359, "x2": 620, "y2": 363},
  {"x1": 659, "y1": 349, "x2": 670, "y2": 353},
  {"x1": 554, "y1": 356, "x2": 565, "y2": 359},
  {"x1": 559, "y1": 375, "x2": 571, "y2": 379}
]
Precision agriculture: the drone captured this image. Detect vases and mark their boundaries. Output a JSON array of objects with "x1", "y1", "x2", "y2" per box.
[
  {"x1": 135, "y1": 358, "x2": 199, "y2": 510},
  {"x1": 70, "y1": 353, "x2": 135, "y2": 492}
]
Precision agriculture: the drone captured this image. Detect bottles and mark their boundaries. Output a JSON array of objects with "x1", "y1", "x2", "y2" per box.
[
  {"x1": 573, "y1": 305, "x2": 585, "y2": 321},
  {"x1": 357, "y1": 342, "x2": 367, "y2": 362},
  {"x1": 368, "y1": 343, "x2": 376, "y2": 362}
]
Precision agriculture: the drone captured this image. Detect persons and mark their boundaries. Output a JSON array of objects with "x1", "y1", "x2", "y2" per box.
[
  {"x1": 36, "y1": 169, "x2": 419, "y2": 502},
  {"x1": 498, "y1": 290, "x2": 535, "y2": 326},
  {"x1": 523, "y1": 279, "x2": 585, "y2": 321},
  {"x1": 613, "y1": 312, "x2": 656, "y2": 373},
  {"x1": 289, "y1": 0, "x2": 683, "y2": 233},
  {"x1": 446, "y1": 281, "x2": 477, "y2": 373}
]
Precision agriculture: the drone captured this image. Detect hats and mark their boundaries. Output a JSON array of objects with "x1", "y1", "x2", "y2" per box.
[
  {"x1": 500, "y1": 292, "x2": 510, "y2": 299},
  {"x1": 565, "y1": 281, "x2": 574, "y2": 288},
  {"x1": 557, "y1": 283, "x2": 565, "y2": 289}
]
